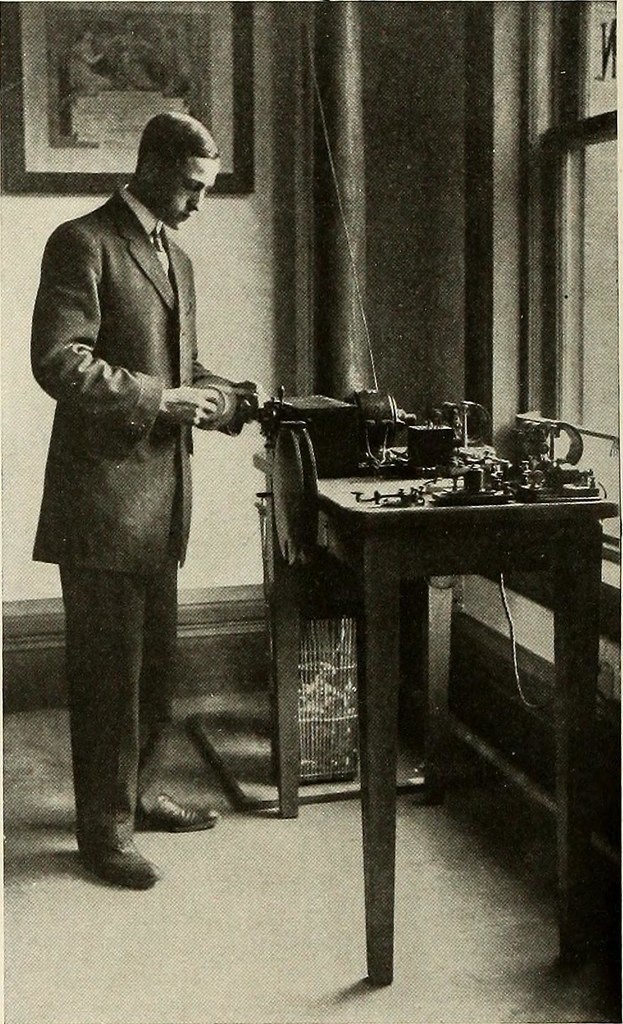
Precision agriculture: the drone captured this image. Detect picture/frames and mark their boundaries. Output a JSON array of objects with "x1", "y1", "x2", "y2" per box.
[{"x1": 0, "y1": 0, "x2": 254, "y2": 200}]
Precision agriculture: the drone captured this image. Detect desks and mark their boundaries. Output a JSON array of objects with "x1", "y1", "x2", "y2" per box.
[{"x1": 255, "y1": 454, "x2": 623, "y2": 987}]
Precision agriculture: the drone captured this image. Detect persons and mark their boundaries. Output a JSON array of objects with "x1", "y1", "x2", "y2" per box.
[{"x1": 30, "y1": 113, "x2": 270, "y2": 891}]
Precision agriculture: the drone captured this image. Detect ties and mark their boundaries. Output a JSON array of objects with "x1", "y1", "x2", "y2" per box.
[{"x1": 150, "y1": 227, "x2": 170, "y2": 279}]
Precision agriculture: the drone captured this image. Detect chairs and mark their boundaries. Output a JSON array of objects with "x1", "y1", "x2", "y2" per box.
[{"x1": 270, "y1": 419, "x2": 455, "y2": 819}]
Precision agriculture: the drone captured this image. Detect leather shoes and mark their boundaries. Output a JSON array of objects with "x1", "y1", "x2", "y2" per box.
[
  {"x1": 91, "y1": 850, "x2": 160, "y2": 888},
  {"x1": 139, "y1": 792, "x2": 221, "y2": 834}
]
